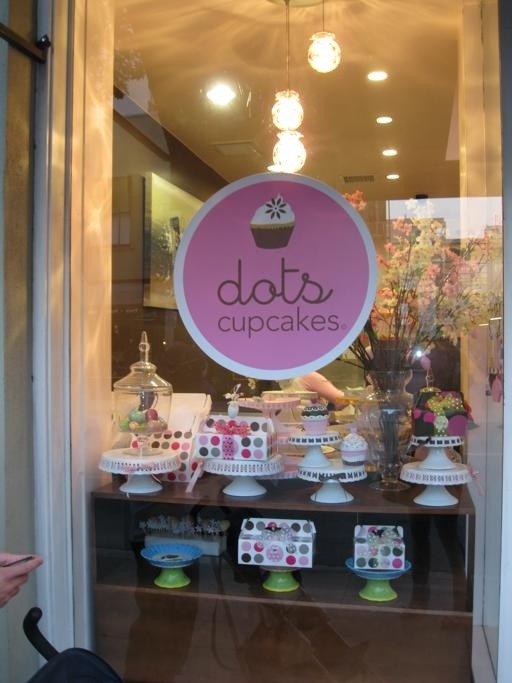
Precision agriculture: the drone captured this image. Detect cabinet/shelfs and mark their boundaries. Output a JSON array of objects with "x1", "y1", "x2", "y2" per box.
[{"x1": 88, "y1": 478, "x2": 475, "y2": 682}]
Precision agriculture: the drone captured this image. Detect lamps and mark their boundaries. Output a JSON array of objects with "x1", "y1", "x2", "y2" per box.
[
  {"x1": 270, "y1": 132, "x2": 308, "y2": 173},
  {"x1": 305, "y1": 30, "x2": 343, "y2": 74},
  {"x1": 270, "y1": 90, "x2": 306, "y2": 130}
]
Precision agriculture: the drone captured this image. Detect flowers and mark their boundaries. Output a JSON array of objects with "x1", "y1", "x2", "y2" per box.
[
  {"x1": 411, "y1": 387, "x2": 481, "y2": 438},
  {"x1": 330, "y1": 189, "x2": 503, "y2": 490}
]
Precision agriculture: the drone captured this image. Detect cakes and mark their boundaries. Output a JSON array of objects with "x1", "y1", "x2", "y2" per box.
[
  {"x1": 341, "y1": 431, "x2": 368, "y2": 465},
  {"x1": 300, "y1": 405, "x2": 329, "y2": 436},
  {"x1": 250, "y1": 191, "x2": 297, "y2": 249}
]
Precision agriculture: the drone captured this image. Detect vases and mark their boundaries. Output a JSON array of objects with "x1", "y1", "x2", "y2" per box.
[{"x1": 361, "y1": 364, "x2": 415, "y2": 494}]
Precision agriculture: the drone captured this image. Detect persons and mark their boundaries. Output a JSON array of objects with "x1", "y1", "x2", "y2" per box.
[
  {"x1": 0, "y1": 551, "x2": 44, "y2": 609},
  {"x1": 398, "y1": 334, "x2": 471, "y2": 628},
  {"x1": 298, "y1": 371, "x2": 349, "y2": 410}
]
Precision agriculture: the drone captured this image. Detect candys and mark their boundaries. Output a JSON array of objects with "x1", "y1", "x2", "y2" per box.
[{"x1": 120, "y1": 409, "x2": 167, "y2": 434}]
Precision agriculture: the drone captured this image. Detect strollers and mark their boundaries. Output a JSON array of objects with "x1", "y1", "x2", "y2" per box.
[{"x1": 193, "y1": 486, "x2": 373, "y2": 680}]
